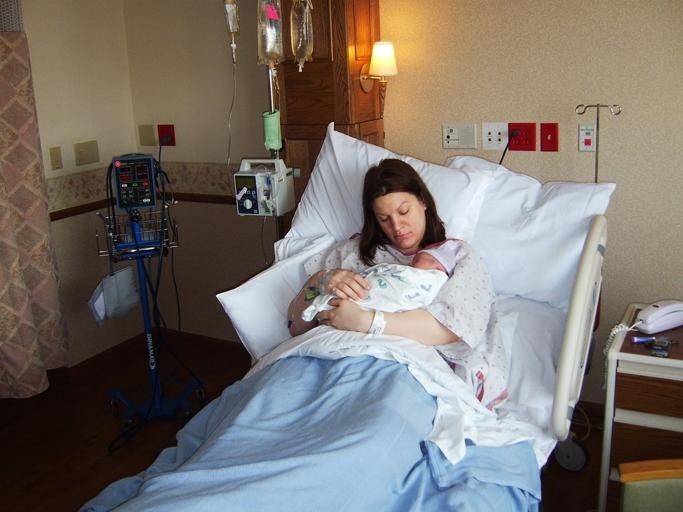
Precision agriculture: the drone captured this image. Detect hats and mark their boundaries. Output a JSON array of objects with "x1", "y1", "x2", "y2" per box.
[{"x1": 416, "y1": 246, "x2": 457, "y2": 278}]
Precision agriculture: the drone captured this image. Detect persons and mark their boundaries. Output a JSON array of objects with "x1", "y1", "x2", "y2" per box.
[
  {"x1": 301, "y1": 238, "x2": 465, "y2": 323},
  {"x1": 288, "y1": 159, "x2": 493, "y2": 361}
]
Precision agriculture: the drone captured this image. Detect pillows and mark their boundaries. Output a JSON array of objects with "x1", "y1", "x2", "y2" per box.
[
  {"x1": 442, "y1": 155, "x2": 616, "y2": 309},
  {"x1": 272, "y1": 122, "x2": 490, "y2": 264},
  {"x1": 216, "y1": 231, "x2": 337, "y2": 364}
]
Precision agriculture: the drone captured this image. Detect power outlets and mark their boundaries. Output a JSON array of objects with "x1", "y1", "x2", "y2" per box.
[
  {"x1": 442, "y1": 123, "x2": 476, "y2": 149},
  {"x1": 508, "y1": 123, "x2": 536, "y2": 151},
  {"x1": 481, "y1": 122, "x2": 508, "y2": 152}
]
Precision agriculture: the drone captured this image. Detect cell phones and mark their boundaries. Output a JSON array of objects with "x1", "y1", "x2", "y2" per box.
[
  {"x1": 652, "y1": 336, "x2": 672, "y2": 350},
  {"x1": 651, "y1": 350, "x2": 670, "y2": 358}
]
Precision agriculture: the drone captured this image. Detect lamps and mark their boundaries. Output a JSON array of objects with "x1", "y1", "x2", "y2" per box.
[{"x1": 359, "y1": 41, "x2": 398, "y2": 119}]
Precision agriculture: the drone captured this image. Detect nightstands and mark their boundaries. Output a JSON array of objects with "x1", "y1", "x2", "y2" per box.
[{"x1": 598, "y1": 302, "x2": 682, "y2": 511}]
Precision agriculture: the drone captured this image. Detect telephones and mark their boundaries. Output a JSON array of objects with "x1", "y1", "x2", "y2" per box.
[{"x1": 634, "y1": 299, "x2": 683, "y2": 335}]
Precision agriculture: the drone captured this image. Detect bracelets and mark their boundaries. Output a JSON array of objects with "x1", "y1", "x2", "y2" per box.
[{"x1": 368, "y1": 308, "x2": 386, "y2": 335}]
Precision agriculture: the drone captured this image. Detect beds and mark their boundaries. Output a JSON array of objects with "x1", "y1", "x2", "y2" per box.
[{"x1": 77, "y1": 122, "x2": 618, "y2": 512}]
